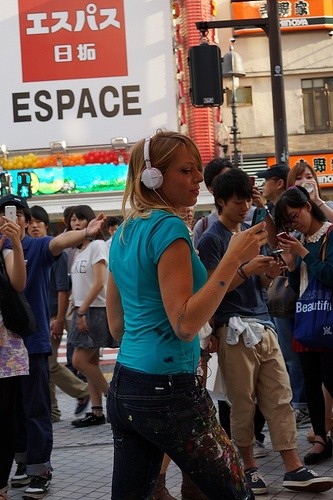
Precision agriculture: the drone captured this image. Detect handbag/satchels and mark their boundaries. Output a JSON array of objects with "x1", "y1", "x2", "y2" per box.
[{"x1": 266, "y1": 262, "x2": 300, "y2": 317}]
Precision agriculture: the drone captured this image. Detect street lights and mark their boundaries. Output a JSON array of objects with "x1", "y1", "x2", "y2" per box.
[{"x1": 221, "y1": 43, "x2": 247, "y2": 168}]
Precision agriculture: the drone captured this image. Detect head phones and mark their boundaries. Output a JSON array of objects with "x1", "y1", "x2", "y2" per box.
[{"x1": 141, "y1": 138, "x2": 163, "y2": 190}]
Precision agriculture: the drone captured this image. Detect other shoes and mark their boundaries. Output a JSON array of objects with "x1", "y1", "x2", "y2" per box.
[
  {"x1": 252, "y1": 439, "x2": 266, "y2": 458},
  {"x1": 0, "y1": 484, "x2": 9, "y2": 500},
  {"x1": 303, "y1": 440, "x2": 331, "y2": 465},
  {"x1": 294, "y1": 408, "x2": 312, "y2": 429},
  {"x1": 74, "y1": 395, "x2": 90, "y2": 414},
  {"x1": 72, "y1": 412, "x2": 107, "y2": 427}
]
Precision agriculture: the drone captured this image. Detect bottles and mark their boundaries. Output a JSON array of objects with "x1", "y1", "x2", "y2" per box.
[{"x1": 301, "y1": 182, "x2": 314, "y2": 192}]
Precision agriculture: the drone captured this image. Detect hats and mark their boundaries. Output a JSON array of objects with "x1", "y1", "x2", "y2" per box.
[
  {"x1": 256, "y1": 165, "x2": 290, "y2": 182},
  {"x1": 0, "y1": 194, "x2": 32, "y2": 220}
]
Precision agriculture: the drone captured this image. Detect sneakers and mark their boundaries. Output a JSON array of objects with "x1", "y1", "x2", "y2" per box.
[
  {"x1": 11, "y1": 461, "x2": 31, "y2": 488},
  {"x1": 22, "y1": 472, "x2": 52, "y2": 500},
  {"x1": 244, "y1": 466, "x2": 268, "y2": 494},
  {"x1": 281, "y1": 466, "x2": 333, "y2": 492}
]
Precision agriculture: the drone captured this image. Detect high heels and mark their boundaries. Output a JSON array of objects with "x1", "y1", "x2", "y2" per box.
[{"x1": 306, "y1": 422, "x2": 333, "y2": 442}]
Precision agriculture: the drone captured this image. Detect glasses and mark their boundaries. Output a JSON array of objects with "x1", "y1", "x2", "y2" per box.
[{"x1": 283, "y1": 208, "x2": 302, "y2": 232}]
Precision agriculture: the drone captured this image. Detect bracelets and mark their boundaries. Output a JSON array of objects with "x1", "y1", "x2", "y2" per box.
[
  {"x1": 237, "y1": 270, "x2": 245, "y2": 279},
  {"x1": 240, "y1": 267, "x2": 249, "y2": 280},
  {"x1": 77, "y1": 312, "x2": 86, "y2": 318},
  {"x1": 84, "y1": 228, "x2": 95, "y2": 240},
  {"x1": 319, "y1": 203, "x2": 324, "y2": 207}
]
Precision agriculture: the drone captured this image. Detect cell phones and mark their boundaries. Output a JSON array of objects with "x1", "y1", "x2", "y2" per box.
[
  {"x1": 5, "y1": 206, "x2": 16, "y2": 223},
  {"x1": 250, "y1": 177, "x2": 255, "y2": 186},
  {"x1": 267, "y1": 249, "x2": 283, "y2": 258},
  {"x1": 277, "y1": 231, "x2": 291, "y2": 241},
  {"x1": 251, "y1": 207, "x2": 266, "y2": 234}
]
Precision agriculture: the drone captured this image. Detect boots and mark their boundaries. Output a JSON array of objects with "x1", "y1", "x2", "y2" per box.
[{"x1": 153, "y1": 472, "x2": 178, "y2": 500}]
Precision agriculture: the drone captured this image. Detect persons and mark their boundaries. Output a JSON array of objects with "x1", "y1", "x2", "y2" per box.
[
  {"x1": 0, "y1": 195, "x2": 122, "y2": 500},
  {"x1": 151, "y1": 453, "x2": 176, "y2": 500},
  {"x1": 193, "y1": 157, "x2": 333, "y2": 466},
  {"x1": 198, "y1": 168, "x2": 333, "y2": 494},
  {"x1": 106, "y1": 131, "x2": 268, "y2": 500}
]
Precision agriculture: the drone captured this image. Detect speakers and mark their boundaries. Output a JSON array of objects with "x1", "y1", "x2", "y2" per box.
[{"x1": 187, "y1": 45, "x2": 224, "y2": 108}]
